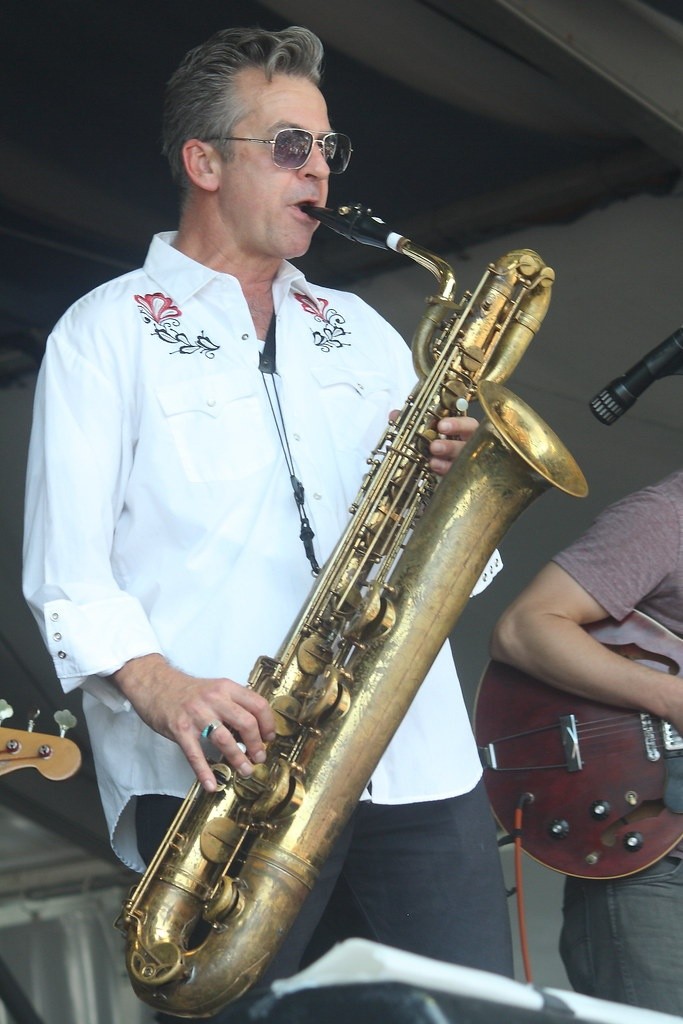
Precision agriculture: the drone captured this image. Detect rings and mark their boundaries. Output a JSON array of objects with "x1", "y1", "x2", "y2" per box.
[{"x1": 201, "y1": 720, "x2": 222, "y2": 738}]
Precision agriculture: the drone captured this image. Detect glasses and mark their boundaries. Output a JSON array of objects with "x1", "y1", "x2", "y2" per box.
[{"x1": 201, "y1": 128, "x2": 353, "y2": 174}]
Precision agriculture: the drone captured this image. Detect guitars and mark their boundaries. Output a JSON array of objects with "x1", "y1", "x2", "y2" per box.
[
  {"x1": 473, "y1": 603, "x2": 682, "y2": 881},
  {"x1": 0, "y1": 695, "x2": 83, "y2": 785}
]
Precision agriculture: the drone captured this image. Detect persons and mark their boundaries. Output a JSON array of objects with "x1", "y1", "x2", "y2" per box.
[
  {"x1": 492, "y1": 467, "x2": 683, "y2": 1018},
  {"x1": 22, "y1": 26, "x2": 515, "y2": 1024}
]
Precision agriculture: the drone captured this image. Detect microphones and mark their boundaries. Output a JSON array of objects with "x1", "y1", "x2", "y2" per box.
[{"x1": 590, "y1": 327, "x2": 683, "y2": 426}]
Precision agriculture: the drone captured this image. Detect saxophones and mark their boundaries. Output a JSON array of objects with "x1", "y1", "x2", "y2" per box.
[{"x1": 110, "y1": 198, "x2": 592, "y2": 1022}]
